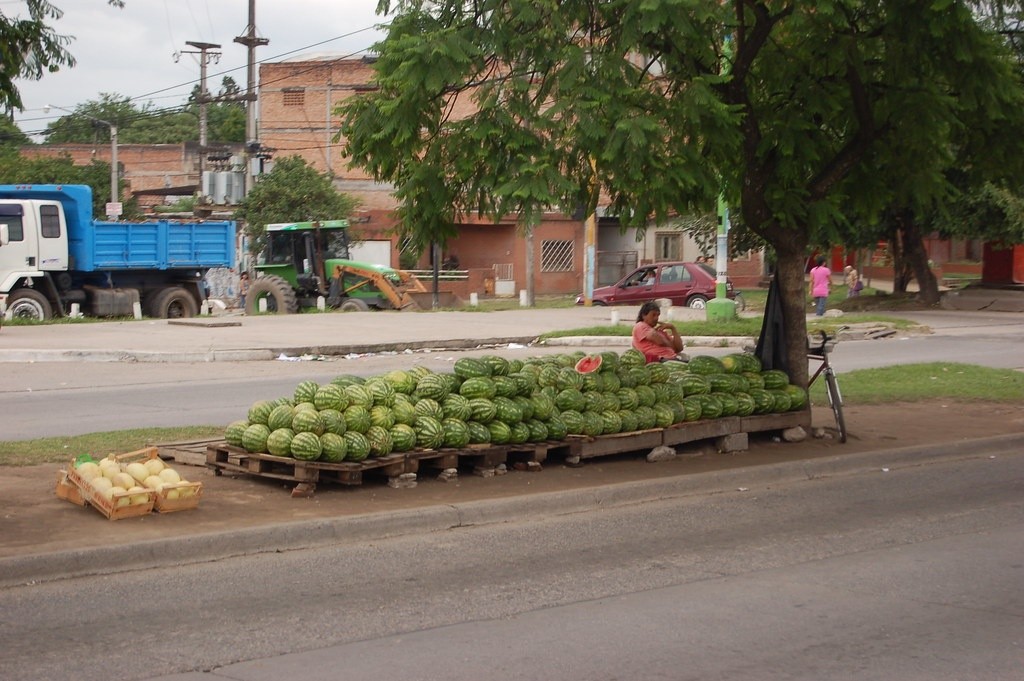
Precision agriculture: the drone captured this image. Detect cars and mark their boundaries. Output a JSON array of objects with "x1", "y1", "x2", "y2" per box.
[
  {"x1": 574, "y1": 262, "x2": 735, "y2": 309},
  {"x1": 743, "y1": 326, "x2": 850, "y2": 443}
]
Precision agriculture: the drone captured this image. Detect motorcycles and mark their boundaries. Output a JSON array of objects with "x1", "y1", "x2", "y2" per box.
[{"x1": 733, "y1": 291, "x2": 746, "y2": 312}]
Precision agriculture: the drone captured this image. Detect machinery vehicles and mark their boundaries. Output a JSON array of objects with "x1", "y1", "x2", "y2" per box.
[{"x1": 244, "y1": 219, "x2": 471, "y2": 315}]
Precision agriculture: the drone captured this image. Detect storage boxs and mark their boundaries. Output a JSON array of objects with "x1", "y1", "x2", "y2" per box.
[{"x1": 55, "y1": 445, "x2": 204, "y2": 522}]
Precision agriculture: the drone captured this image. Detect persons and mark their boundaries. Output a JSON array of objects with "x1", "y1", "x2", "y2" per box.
[
  {"x1": 631, "y1": 301, "x2": 684, "y2": 365},
  {"x1": 845, "y1": 265, "x2": 858, "y2": 298},
  {"x1": 808, "y1": 256, "x2": 833, "y2": 316},
  {"x1": 631, "y1": 270, "x2": 655, "y2": 286}
]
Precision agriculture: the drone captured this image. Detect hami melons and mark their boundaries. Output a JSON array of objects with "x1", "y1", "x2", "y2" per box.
[{"x1": 75, "y1": 457, "x2": 196, "y2": 508}]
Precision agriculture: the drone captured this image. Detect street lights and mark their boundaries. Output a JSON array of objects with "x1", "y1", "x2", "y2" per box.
[{"x1": 43, "y1": 103, "x2": 119, "y2": 220}]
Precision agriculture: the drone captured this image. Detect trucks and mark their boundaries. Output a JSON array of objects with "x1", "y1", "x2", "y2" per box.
[{"x1": 0, "y1": 184, "x2": 237, "y2": 320}]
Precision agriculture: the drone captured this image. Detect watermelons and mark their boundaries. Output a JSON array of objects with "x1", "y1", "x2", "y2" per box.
[{"x1": 222, "y1": 348, "x2": 807, "y2": 461}]
